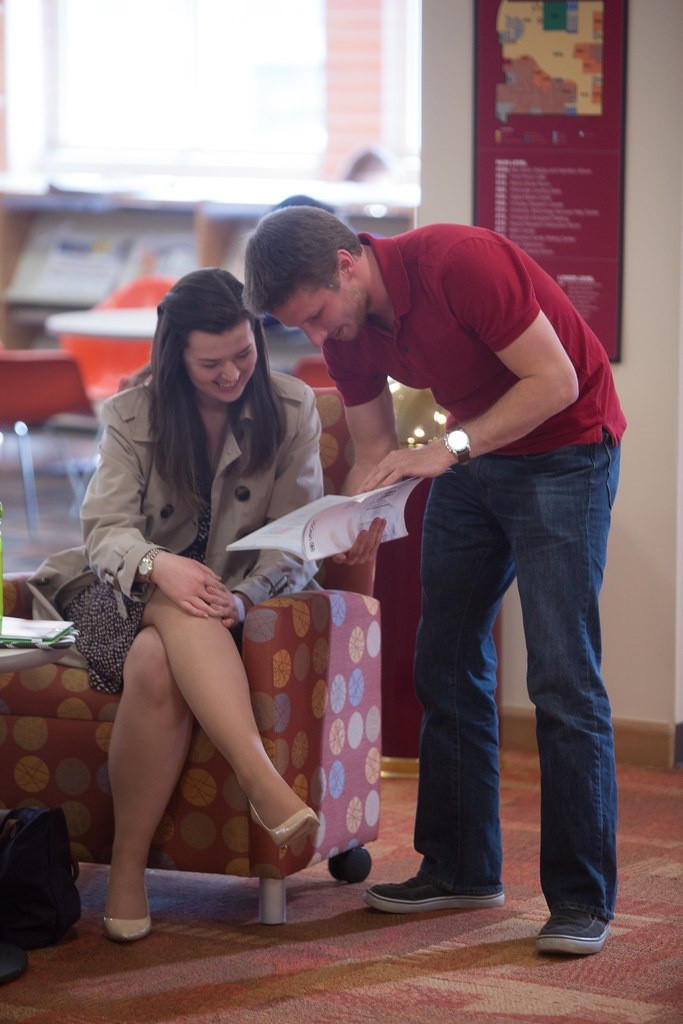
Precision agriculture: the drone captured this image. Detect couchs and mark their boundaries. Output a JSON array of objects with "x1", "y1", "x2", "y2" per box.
[
  {"x1": 0, "y1": 364, "x2": 383, "y2": 925},
  {"x1": 282, "y1": 354, "x2": 502, "y2": 759}
]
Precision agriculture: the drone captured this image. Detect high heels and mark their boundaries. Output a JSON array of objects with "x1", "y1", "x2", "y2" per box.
[
  {"x1": 248, "y1": 797, "x2": 320, "y2": 848},
  {"x1": 103, "y1": 866, "x2": 151, "y2": 941}
]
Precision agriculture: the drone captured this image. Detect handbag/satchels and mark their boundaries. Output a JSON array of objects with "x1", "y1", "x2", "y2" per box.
[{"x1": 0, "y1": 806, "x2": 80, "y2": 948}]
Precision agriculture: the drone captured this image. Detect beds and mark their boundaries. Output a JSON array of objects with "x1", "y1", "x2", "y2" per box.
[{"x1": 59, "y1": 279, "x2": 176, "y2": 402}]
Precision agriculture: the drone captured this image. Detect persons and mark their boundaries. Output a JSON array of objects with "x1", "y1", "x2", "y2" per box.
[
  {"x1": 69, "y1": 268, "x2": 323, "y2": 943},
  {"x1": 242, "y1": 205, "x2": 628, "y2": 954}
]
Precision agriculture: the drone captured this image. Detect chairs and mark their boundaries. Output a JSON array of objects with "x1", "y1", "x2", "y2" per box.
[{"x1": 0, "y1": 341, "x2": 100, "y2": 534}]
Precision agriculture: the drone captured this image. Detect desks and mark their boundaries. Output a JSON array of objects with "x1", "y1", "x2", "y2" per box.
[{"x1": 47, "y1": 308, "x2": 161, "y2": 339}]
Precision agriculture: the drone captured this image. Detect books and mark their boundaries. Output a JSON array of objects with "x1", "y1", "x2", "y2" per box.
[{"x1": 226, "y1": 477, "x2": 425, "y2": 561}]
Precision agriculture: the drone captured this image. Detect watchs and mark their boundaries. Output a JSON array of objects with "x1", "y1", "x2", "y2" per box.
[
  {"x1": 138, "y1": 549, "x2": 165, "y2": 584},
  {"x1": 444, "y1": 429, "x2": 472, "y2": 462}
]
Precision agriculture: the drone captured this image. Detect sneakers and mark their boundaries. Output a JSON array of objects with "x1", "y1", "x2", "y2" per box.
[
  {"x1": 363, "y1": 876, "x2": 502, "y2": 913},
  {"x1": 535, "y1": 909, "x2": 610, "y2": 953}
]
